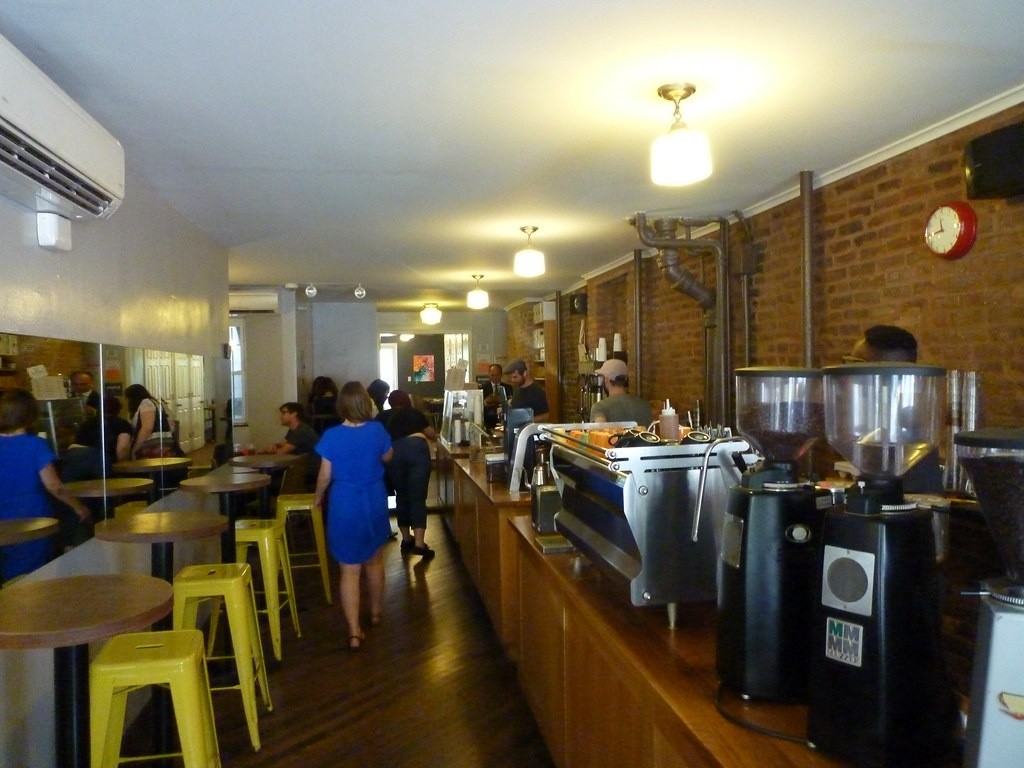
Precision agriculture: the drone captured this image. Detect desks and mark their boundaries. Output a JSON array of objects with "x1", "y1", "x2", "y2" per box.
[
  {"x1": 179, "y1": 474, "x2": 275, "y2": 681},
  {"x1": 0, "y1": 572, "x2": 175, "y2": 768},
  {"x1": 229, "y1": 452, "x2": 302, "y2": 519},
  {"x1": 64, "y1": 478, "x2": 154, "y2": 521},
  {"x1": 0, "y1": 517, "x2": 61, "y2": 546},
  {"x1": 113, "y1": 458, "x2": 192, "y2": 505},
  {"x1": 95, "y1": 511, "x2": 230, "y2": 768}
]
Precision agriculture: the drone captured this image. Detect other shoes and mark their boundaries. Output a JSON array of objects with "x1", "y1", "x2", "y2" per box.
[
  {"x1": 412, "y1": 544, "x2": 436, "y2": 556},
  {"x1": 388, "y1": 530, "x2": 399, "y2": 539},
  {"x1": 401, "y1": 538, "x2": 416, "y2": 552}
]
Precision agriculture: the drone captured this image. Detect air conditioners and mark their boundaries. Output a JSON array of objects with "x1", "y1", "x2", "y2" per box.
[
  {"x1": 225, "y1": 289, "x2": 283, "y2": 315},
  {"x1": 0, "y1": 32, "x2": 127, "y2": 221}
]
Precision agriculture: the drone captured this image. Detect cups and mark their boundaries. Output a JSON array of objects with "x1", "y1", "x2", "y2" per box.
[
  {"x1": 594, "y1": 332, "x2": 622, "y2": 362},
  {"x1": 552, "y1": 425, "x2": 713, "y2": 463}
]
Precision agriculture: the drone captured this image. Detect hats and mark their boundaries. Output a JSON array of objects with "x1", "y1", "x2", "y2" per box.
[
  {"x1": 503, "y1": 358, "x2": 525, "y2": 374},
  {"x1": 594, "y1": 360, "x2": 627, "y2": 382}
]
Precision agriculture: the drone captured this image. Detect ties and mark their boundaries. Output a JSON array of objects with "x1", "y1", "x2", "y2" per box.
[{"x1": 495, "y1": 384, "x2": 499, "y2": 396}]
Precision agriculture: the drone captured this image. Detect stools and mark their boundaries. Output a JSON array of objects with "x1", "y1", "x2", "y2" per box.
[
  {"x1": 277, "y1": 493, "x2": 335, "y2": 606},
  {"x1": 206, "y1": 517, "x2": 301, "y2": 662},
  {"x1": 170, "y1": 562, "x2": 274, "y2": 751},
  {"x1": 90, "y1": 630, "x2": 221, "y2": 768}
]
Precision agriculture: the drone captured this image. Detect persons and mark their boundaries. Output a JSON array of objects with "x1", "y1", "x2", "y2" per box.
[
  {"x1": 0, "y1": 388, "x2": 92, "y2": 588},
  {"x1": 839, "y1": 325, "x2": 946, "y2": 493},
  {"x1": 257, "y1": 402, "x2": 321, "y2": 496},
  {"x1": 367, "y1": 378, "x2": 389, "y2": 413},
  {"x1": 373, "y1": 390, "x2": 436, "y2": 555},
  {"x1": 590, "y1": 359, "x2": 653, "y2": 431},
  {"x1": 67, "y1": 371, "x2": 107, "y2": 419},
  {"x1": 479, "y1": 364, "x2": 513, "y2": 433},
  {"x1": 306, "y1": 377, "x2": 345, "y2": 438},
  {"x1": 312, "y1": 381, "x2": 393, "y2": 650},
  {"x1": 124, "y1": 384, "x2": 188, "y2": 502},
  {"x1": 504, "y1": 359, "x2": 550, "y2": 429},
  {"x1": 58, "y1": 396, "x2": 134, "y2": 529}
]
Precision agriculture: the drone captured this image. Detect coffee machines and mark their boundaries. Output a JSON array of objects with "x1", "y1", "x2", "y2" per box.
[{"x1": 716, "y1": 361, "x2": 1022, "y2": 768}]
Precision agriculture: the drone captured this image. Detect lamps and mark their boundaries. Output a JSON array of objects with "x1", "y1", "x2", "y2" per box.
[
  {"x1": 420, "y1": 303, "x2": 442, "y2": 325},
  {"x1": 467, "y1": 275, "x2": 489, "y2": 309},
  {"x1": 649, "y1": 83, "x2": 712, "y2": 187},
  {"x1": 514, "y1": 225, "x2": 544, "y2": 278}
]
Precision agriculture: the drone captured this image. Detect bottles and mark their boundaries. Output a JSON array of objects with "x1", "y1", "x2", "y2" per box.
[
  {"x1": 658, "y1": 399, "x2": 678, "y2": 441},
  {"x1": 463, "y1": 419, "x2": 470, "y2": 445},
  {"x1": 453, "y1": 415, "x2": 462, "y2": 444}
]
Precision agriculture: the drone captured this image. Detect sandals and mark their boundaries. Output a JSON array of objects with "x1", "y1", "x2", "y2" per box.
[
  {"x1": 349, "y1": 634, "x2": 365, "y2": 652},
  {"x1": 372, "y1": 605, "x2": 383, "y2": 625}
]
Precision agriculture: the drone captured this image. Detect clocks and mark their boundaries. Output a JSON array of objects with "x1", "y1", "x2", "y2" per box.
[{"x1": 923, "y1": 201, "x2": 977, "y2": 260}]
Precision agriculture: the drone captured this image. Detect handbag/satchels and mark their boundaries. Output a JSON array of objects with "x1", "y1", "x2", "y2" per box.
[{"x1": 136, "y1": 436, "x2": 178, "y2": 459}]
